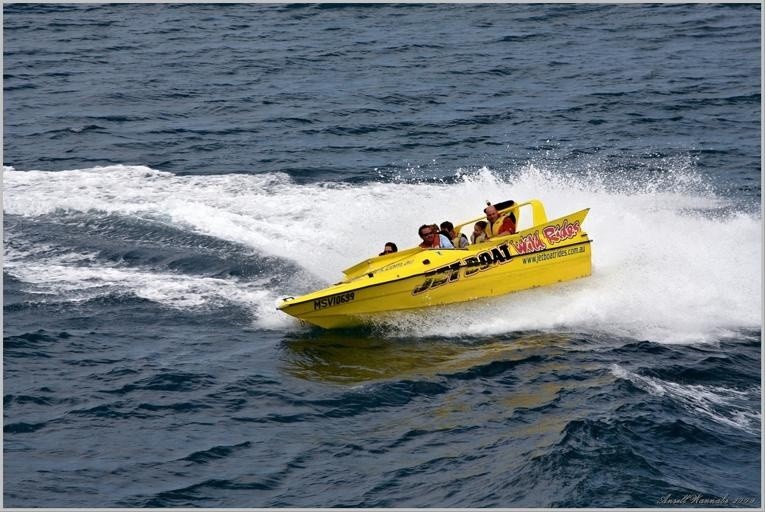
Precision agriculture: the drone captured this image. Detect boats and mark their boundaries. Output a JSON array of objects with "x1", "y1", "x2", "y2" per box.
[{"x1": 273, "y1": 195, "x2": 598, "y2": 336}]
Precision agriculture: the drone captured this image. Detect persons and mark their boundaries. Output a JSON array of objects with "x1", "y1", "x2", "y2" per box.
[
  {"x1": 419, "y1": 225, "x2": 454, "y2": 249},
  {"x1": 471, "y1": 221, "x2": 487, "y2": 244},
  {"x1": 384, "y1": 243, "x2": 397, "y2": 254},
  {"x1": 483, "y1": 205, "x2": 515, "y2": 236},
  {"x1": 440, "y1": 222, "x2": 468, "y2": 248}
]
